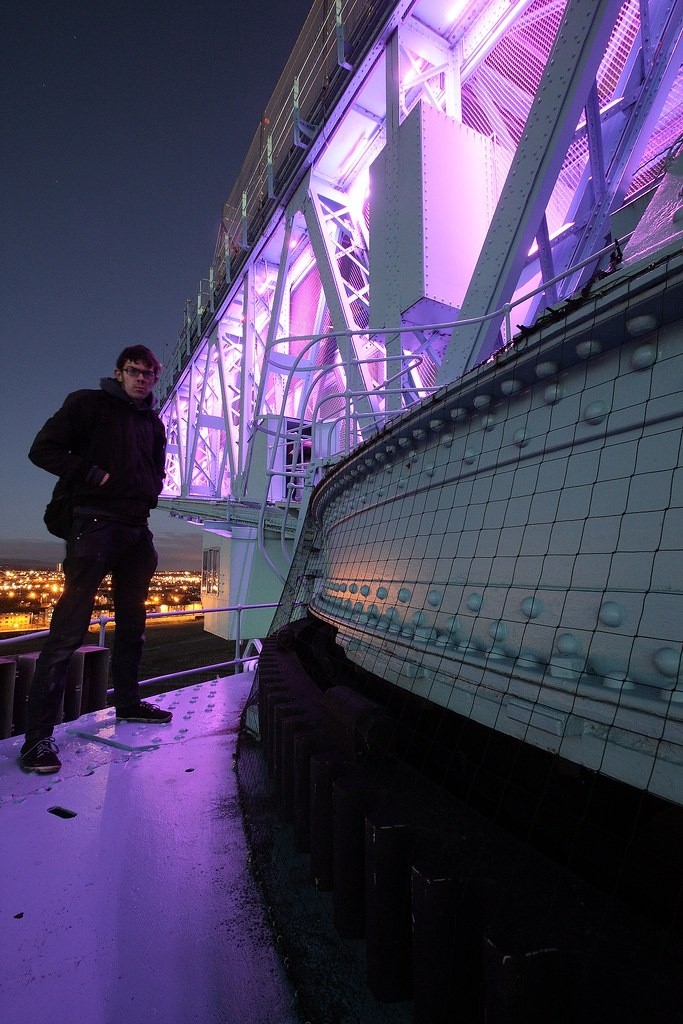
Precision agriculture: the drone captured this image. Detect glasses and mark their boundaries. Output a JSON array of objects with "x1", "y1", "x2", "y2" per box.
[{"x1": 122, "y1": 367, "x2": 156, "y2": 377}]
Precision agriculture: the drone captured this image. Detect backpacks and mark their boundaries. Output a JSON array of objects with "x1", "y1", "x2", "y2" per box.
[{"x1": 44, "y1": 392, "x2": 161, "y2": 536}]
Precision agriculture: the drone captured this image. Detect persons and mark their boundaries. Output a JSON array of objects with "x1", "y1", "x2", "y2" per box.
[{"x1": 17, "y1": 344, "x2": 173, "y2": 773}]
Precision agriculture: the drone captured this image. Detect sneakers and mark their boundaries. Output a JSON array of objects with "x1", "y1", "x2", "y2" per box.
[
  {"x1": 20, "y1": 737, "x2": 62, "y2": 773},
  {"x1": 115, "y1": 703, "x2": 172, "y2": 724}
]
